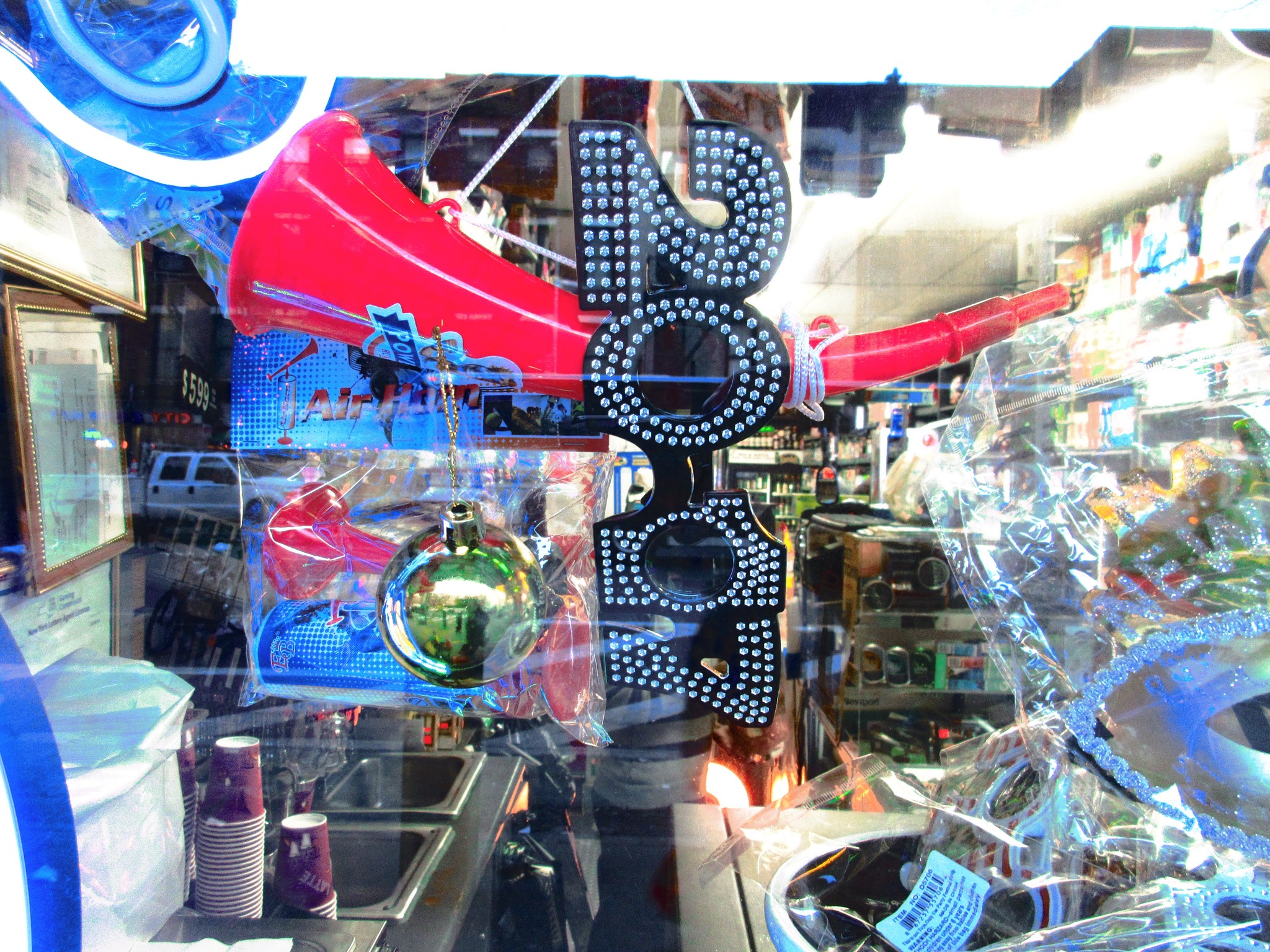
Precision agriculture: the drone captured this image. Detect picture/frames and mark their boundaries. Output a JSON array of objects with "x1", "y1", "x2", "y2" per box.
[
  {"x1": 0, "y1": 285, "x2": 134, "y2": 598},
  {"x1": 0, "y1": 28, "x2": 149, "y2": 318}
]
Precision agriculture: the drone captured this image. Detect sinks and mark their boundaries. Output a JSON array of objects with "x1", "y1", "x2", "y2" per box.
[
  {"x1": 314, "y1": 750, "x2": 489, "y2": 821},
  {"x1": 261, "y1": 823, "x2": 457, "y2": 924}
]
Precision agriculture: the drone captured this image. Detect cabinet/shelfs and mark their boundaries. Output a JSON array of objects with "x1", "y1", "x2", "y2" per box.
[{"x1": 719, "y1": 271, "x2": 1270, "y2": 873}]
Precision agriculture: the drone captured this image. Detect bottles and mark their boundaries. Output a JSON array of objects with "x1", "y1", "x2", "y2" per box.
[
  {"x1": 726, "y1": 425, "x2": 871, "y2": 534},
  {"x1": 887, "y1": 408, "x2": 907, "y2": 468}
]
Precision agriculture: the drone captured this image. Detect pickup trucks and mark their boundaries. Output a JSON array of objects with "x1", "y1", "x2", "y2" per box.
[{"x1": 43, "y1": 452, "x2": 303, "y2": 527}]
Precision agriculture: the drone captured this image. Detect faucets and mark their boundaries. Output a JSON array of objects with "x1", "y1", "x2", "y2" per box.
[
  {"x1": 328, "y1": 711, "x2": 350, "y2": 765},
  {"x1": 267, "y1": 767, "x2": 297, "y2": 828}
]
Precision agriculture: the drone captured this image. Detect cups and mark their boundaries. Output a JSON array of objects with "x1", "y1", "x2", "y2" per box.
[
  {"x1": 177, "y1": 702, "x2": 198, "y2": 902},
  {"x1": 296, "y1": 776, "x2": 314, "y2": 812},
  {"x1": 197, "y1": 735, "x2": 266, "y2": 919},
  {"x1": 274, "y1": 813, "x2": 339, "y2": 921}
]
